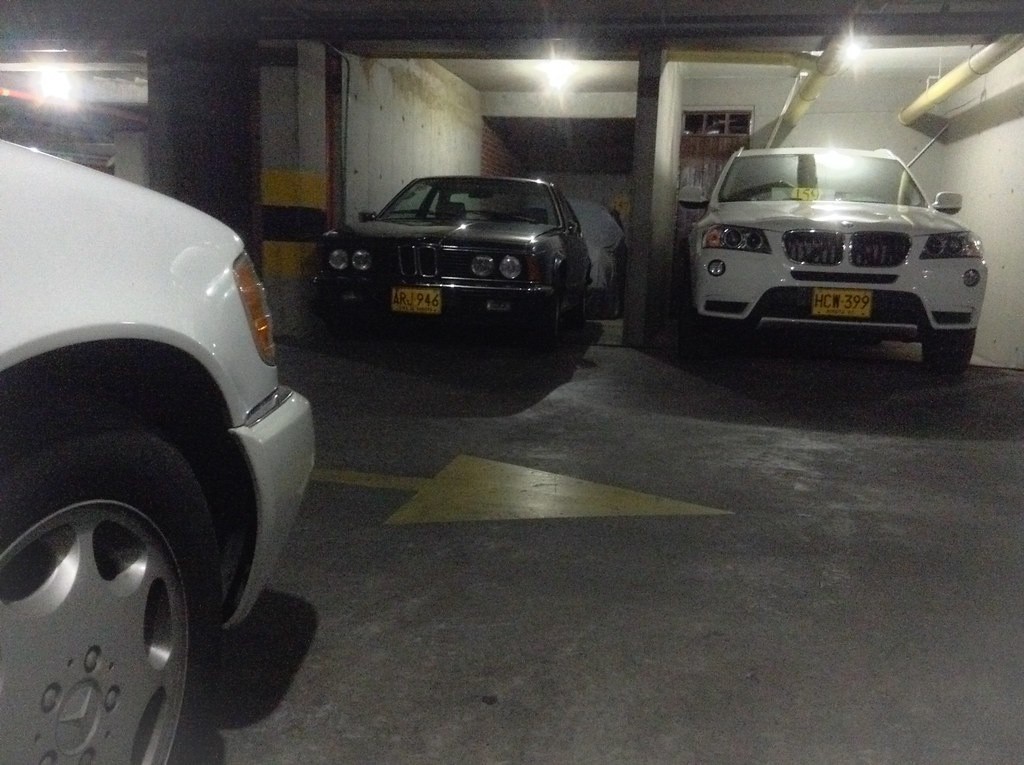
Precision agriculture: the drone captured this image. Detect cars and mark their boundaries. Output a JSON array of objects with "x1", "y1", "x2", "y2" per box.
[
  {"x1": 679, "y1": 147, "x2": 988, "y2": 377},
  {"x1": 314, "y1": 176, "x2": 591, "y2": 355},
  {"x1": 0, "y1": 138, "x2": 315, "y2": 765}
]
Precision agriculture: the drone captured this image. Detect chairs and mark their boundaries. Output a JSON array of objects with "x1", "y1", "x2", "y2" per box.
[
  {"x1": 435, "y1": 202, "x2": 466, "y2": 221},
  {"x1": 518, "y1": 207, "x2": 547, "y2": 225}
]
